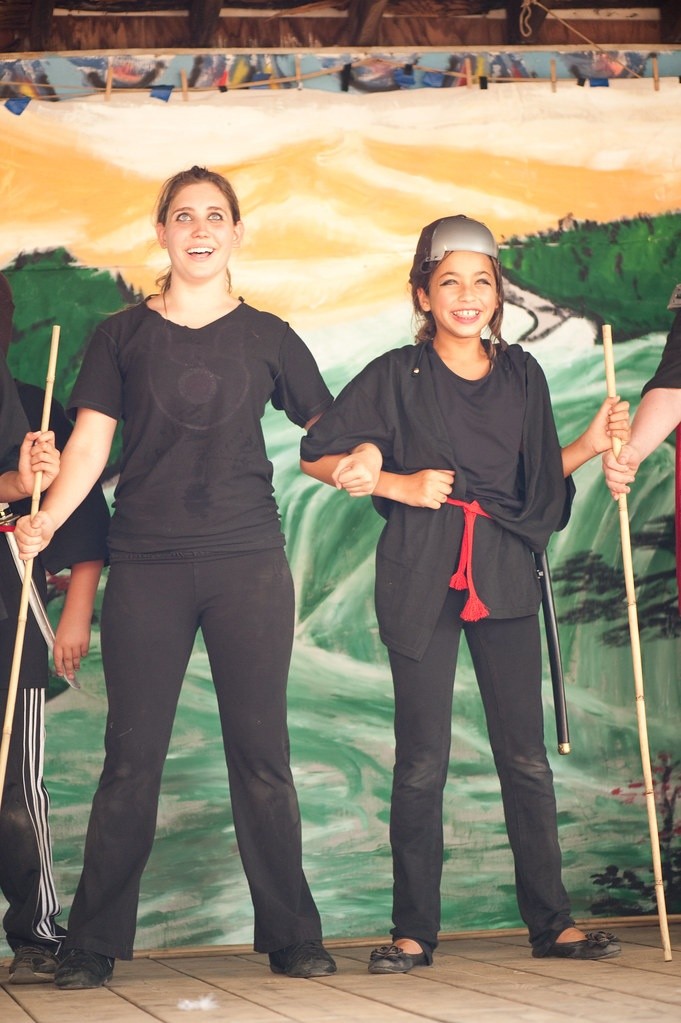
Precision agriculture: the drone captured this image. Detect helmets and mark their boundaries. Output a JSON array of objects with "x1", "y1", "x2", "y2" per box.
[{"x1": 413, "y1": 214, "x2": 500, "y2": 263}]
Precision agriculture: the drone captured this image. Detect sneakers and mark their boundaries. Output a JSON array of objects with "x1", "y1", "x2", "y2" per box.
[
  {"x1": 270, "y1": 943, "x2": 336, "y2": 978},
  {"x1": 54, "y1": 948, "x2": 114, "y2": 990},
  {"x1": 6, "y1": 944, "x2": 58, "y2": 984}
]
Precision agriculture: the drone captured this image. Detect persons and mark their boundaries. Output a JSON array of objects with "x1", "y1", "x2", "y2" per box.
[
  {"x1": 604, "y1": 307, "x2": 680, "y2": 500},
  {"x1": 297, "y1": 216, "x2": 634, "y2": 975},
  {"x1": 1, "y1": 350, "x2": 61, "y2": 501},
  {"x1": 0, "y1": 377, "x2": 112, "y2": 984},
  {"x1": 12, "y1": 165, "x2": 379, "y2": 990}
]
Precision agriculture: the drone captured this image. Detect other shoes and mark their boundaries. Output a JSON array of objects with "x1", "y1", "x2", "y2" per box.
[
  {"x1": 367, "y1": 944, "x2": 427, "y2": 974},
  {"x1": 547, "y1": 930, "x2": 623, "y2": 959}
]
